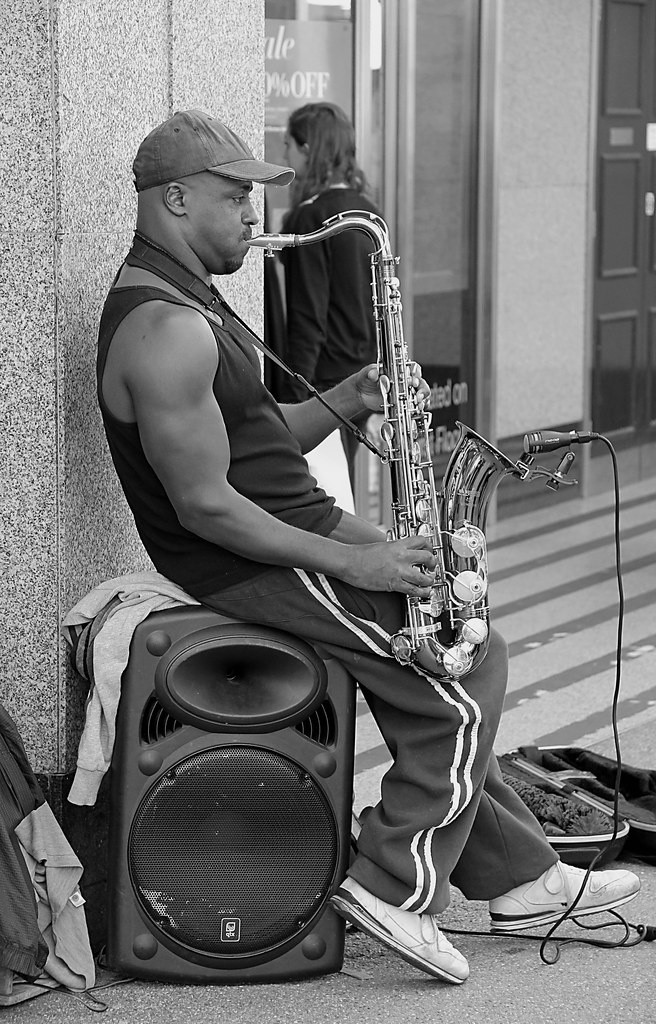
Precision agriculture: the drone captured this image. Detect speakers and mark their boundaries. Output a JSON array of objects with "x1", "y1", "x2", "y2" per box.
[{"x1": 107, "y1": 608, "x2": 357, "y2": 983}]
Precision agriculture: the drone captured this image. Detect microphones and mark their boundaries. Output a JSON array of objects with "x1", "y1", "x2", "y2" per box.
[{"x1": 522, "y1": 431, "x2": 599, "y2": 454}]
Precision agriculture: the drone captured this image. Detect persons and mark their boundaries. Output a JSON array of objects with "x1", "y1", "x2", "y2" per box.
[
  {"x1": 94, "y1": 111, "x2": 639, "y2": 983},
  {"x1": 278, "y1": 103, "x2": 390, "y2": 405}
]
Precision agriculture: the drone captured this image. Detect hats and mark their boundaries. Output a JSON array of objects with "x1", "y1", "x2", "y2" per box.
[{"x1": 133, "y1": 111, "x2": 297, "y2": 194}]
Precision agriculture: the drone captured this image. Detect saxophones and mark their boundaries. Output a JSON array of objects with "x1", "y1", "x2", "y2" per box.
[{"x1": 247, "y1": 207, "x2": 518, "y2": 685}]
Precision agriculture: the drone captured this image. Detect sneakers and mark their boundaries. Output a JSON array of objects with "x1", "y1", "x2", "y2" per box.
[
  {"x1": 332, "y1": 876, "x2": 469, "y2": 986},
  {"x1": 491, "y1": 860, "x2": 642, "y2": 933}
]
